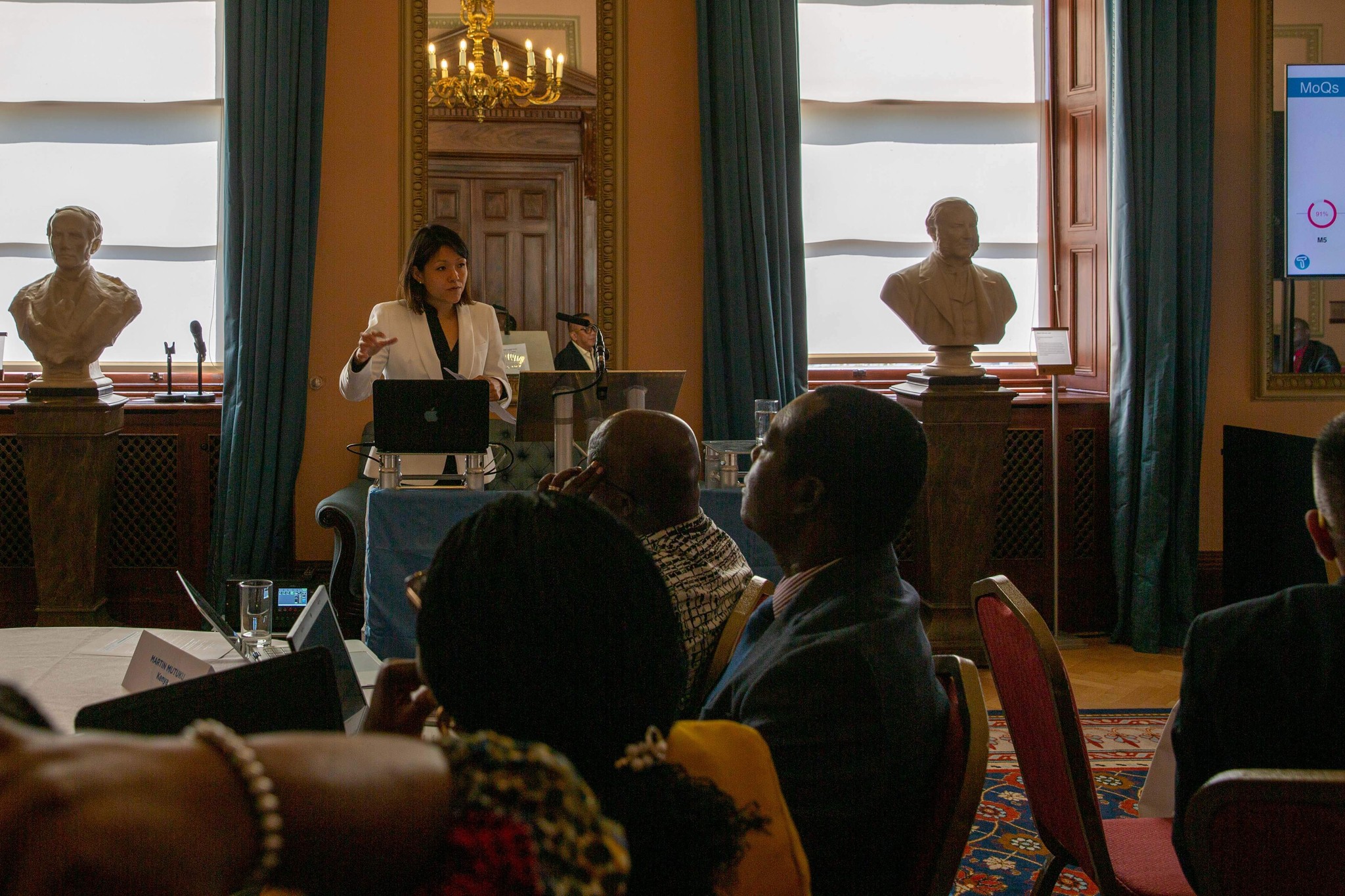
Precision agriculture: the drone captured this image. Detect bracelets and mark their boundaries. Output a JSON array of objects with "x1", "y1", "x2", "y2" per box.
[{"x1": 190, "y1": 719, "x2": 287, "y2": 896}]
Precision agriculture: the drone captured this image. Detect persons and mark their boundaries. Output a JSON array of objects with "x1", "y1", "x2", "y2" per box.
[
  {"x1": 6, "y1": 207, "x2": 142, "y2": 383},
  {"x1": 491, "y1": 305, "x2": 518, "y2": 331},
  {"x1": 554, "y1": 313, "x2": 602, "y2": 371},
  {"x1": 692, "y1": 387, "x2": 964, "y2": 896},
  {"x1": 0, "y1": 689, "x2": 631, "y2": 896},
  {"x1": 405, "y1": 496, "x2": 809, "y2": 896},
  {"x1": 879, "y1": 197, "x2": 1016, "y2": 376},
  {"x1": 338, "y1": 225, "x2": 513, "y2": 492},
  {"x1": 1168, "y1": 418, "x2": 1345, "y2": 896},
  {"x1": 550, "y1": 408, "x2": 756, "y2": 682},
  {"x1": 1293, "y1": 318, "x2": 1341, "y2": 374}
]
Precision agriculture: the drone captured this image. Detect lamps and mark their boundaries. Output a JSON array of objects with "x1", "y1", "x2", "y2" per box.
[{"x1": 427, "y1": 0, "x2": 564, "y2": 123}]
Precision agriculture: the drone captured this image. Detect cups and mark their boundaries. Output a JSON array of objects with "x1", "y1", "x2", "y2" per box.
[
  {"x1": 755, "y1": 399, "x2": 778, "y2": 445},
  {"x1": 239, "y1": 580, "x2": 273, "y2": 647}
]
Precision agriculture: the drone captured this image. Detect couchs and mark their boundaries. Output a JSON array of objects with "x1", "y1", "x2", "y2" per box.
[{"x1": 315, "y1": 419, "x2": 590, "y2": 641}]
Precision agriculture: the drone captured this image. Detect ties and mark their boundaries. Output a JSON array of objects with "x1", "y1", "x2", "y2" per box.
[{"x1": 699, "y1": 596, "x2": 773, "y2": 722}]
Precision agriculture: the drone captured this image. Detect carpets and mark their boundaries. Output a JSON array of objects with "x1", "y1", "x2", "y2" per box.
[{"x1": 946, "y1": 709, "x2": 1172, "y2": 896}]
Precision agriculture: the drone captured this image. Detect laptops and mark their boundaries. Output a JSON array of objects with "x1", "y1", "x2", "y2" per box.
[
  {"x1": 72, "y1": 569, "x2": 389, "y2": 738},
  {"x1": 372, "y1": 379, "x2": 489, "y2": 454}
]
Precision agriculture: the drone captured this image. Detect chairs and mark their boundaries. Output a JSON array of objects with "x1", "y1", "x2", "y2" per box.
[{"x1": 696, "y1": 574, "x2": 1345, "y2": 896}]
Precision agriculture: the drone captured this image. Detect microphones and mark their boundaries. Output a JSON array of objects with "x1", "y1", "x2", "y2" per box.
[
  {"x1": 190, "y1": 320, "x2": 205, "y2": 361},
  {"x1": 492, "y1": 304, "x2": 510, "y2": 334},
  {"x1": 555, "y1": 312, "x2": 605, "y2": 373}
]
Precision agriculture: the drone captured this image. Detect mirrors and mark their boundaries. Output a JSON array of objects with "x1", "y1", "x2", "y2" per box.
[
  {"x1": 1272, "y1": 19, "x2": 1325, "y2": 337},
  {"x1": 1250, "y1": 0, "x2": 1345, "y2": 402}
]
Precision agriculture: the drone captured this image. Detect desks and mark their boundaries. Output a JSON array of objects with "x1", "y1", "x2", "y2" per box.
[{"x1": 365, "y1": 480, "x2": 787, "y2": 662}]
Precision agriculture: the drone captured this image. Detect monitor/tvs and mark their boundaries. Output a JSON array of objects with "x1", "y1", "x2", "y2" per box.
[{"x1": 1283, "y1": 64, "x2": 1345, "y2": 280}]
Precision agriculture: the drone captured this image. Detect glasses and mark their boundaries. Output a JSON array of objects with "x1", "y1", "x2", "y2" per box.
[
  {"x1": 404, "y1": 568, "x2": 426, "y2": 608},
  {"x1": 569, "y1": 326, "x2": 597, "y2": 334}
]
